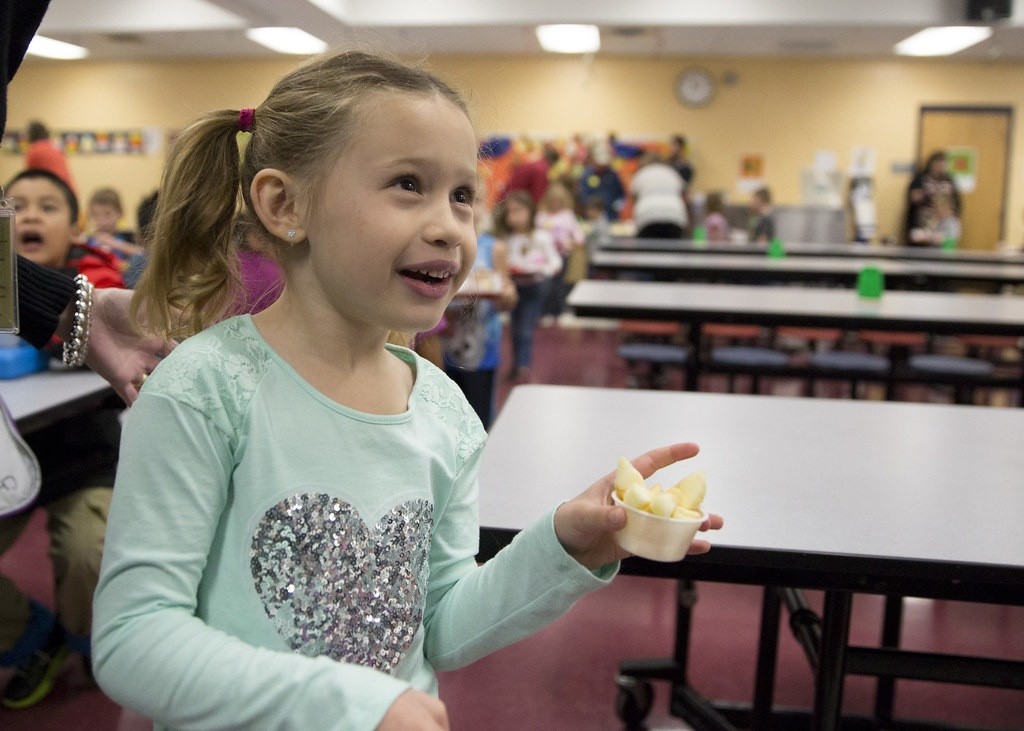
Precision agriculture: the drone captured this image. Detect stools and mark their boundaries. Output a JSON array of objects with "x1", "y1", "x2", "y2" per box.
[
  {"x1": 908, "y1": 356, "x2": 993, "y2": 406},
  {"x1": 615, "y1": 341, "x2": 692, "y2": 393},
  {"x1": 708, "y1": 346, "x2": 793, "y2": 395},
  {"x1": 806, "y1": 351, "x2": 899, "y2": 402}
]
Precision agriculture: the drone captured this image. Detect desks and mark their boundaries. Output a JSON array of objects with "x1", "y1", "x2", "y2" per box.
[
  {"x1": 0, "y1": 360, "x2": 115, "y2": 426},
  {"x1": 474, "y1": 236, "x2": 1024, "y2": 731}
]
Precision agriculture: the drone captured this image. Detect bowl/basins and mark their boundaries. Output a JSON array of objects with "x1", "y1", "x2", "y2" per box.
[{"x1": 612, "y1": 492, "x2": 708, "y2": 564}]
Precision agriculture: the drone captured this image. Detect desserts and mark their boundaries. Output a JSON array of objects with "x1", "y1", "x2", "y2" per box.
[{"x1": 612, "y1": 456, "x2": 709, "y2": 561}]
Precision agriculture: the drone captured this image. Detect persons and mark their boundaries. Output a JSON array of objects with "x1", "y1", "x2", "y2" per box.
[
  {"x1": 583, "y1": 195, "x2": 610, "y2": 278},
  {"x1": 630, "y1": 135, "x2": 696, "y2": 239},
  {"x1": 705, "y1": 191, "x2": 732, "y2": 242},
  {"x1": 748, "y1": 189, "x2": 776, "y2": 245},
  {"x1": 577, "y1": 144, "x2": 626, "y2": 223},
  {"x1": 0, "y1": 168, "x2": 126, "y2": 708},
  {"x1": 26, "y1": 121, "x2": 76, "y2": 193},
  {"x1": 91, "y1": 49, "x2": 724, "y2": 730},
  {"x1": 499, "y1": 190, "x2": 562, "y2": 385},
  {"x1": 436, "y1": 175, "x2": 519, "y2": 430},
  {"x1": 0, "y1": 0, "x2": 216, "y2": 407},
  {"x1": 907, "y1": 152, "x2": 964, "y2": 249},
  {"x1": 497, "y1": 149, "x2": 561, "y2": 204},
  {"x1": 535, "y1": 184, "x2": 585, "y2": 329},
  {"x1": 86, "y1": 188, "x2": 285, "y2": 324}
]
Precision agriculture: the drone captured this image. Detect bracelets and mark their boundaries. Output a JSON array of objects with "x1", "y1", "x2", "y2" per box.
[{"x1": 60, "y1": 273, "x2": 94, "y2": 369}]
